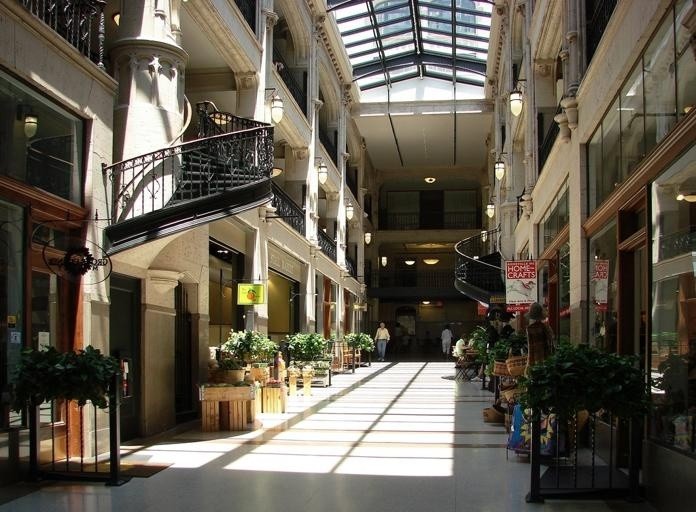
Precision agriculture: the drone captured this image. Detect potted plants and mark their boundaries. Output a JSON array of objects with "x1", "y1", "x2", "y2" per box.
[{"x1": 216, "y1": 329, "x2": 376, "y2": 384}]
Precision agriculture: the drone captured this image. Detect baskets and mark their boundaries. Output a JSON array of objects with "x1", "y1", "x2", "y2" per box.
[
  {"x1": 491, "y1": 359, "x2": 509, "y2": 379},
  {"x1": 505, "y1": 356, "x2": 530, "y2": 376}
]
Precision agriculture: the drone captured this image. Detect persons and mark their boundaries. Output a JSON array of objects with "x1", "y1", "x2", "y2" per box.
[
  {"x1": 522, "y1": 301, "x2": 553, "y2": 376},
  {"x1": 590, "y1": 307, "x2": 618, "y2": 354},
  {"x1": 392, "y1": 322, "x2": 402, "y2": 362},
  {"x1": 439, "y1": 324, "x2": 453, "y2": 360},
  {"x1": 453, "y1": 333, "x2": 473, "y2": 364},
  {"x1": 373, "y1": 322, "x2": 391, "y2": 361}
]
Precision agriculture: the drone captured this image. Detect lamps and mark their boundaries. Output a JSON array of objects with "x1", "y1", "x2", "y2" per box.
[
  {"x1": 112, "y1": 13, "x2": 120, "y2": 27},
  {"x1": 509, "y1": 78, "x2": 527, "y2": 116},
  {"x1": 270, "y1": 168, "x2": 283, "y2": 178},
  {"x1": 364, "y1": 224, "x2": 372, "y2": 245},
  {"x1": 424, "y1": 177, "x2": 436, "y2": 183},
  {"x1": 473, "y1": 255, "x2": 479, "y2": 260},
  {"x1": 486, "y1": 196, "x2": 496, "y2": 218},
  {"x1": 494, "y1": 152, "x2": 508, "y2": 179},
  {"x1": 315, "y1": 156, "x2": 328, "y2": 184},
  {"x1": 423, "y1": 259, "x2": 440, "y2": 265},
  {"x1": 265, "y1": 88, "x2": 284, "y2": 124},
  {"x1": 405, "y1": 261, "x2": 416, "y2": 266},
  {"x1": 381, "y1": 256, "x2": 388, "y2": 267},
  {"x1": 481, "y1": 227, "x2": 488, "y2": 242},
  {"x1": 17, "y1": 104, "x2": 38, "y2": 137},
  {"x1": 344, "y1": 197, "x2": 353, "y2": 220}
]
